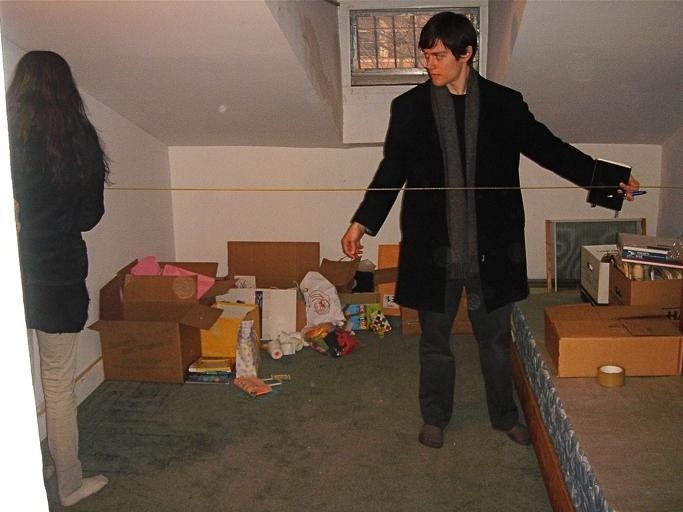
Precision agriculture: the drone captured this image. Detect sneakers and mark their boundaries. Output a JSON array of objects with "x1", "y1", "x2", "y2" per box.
[
  {"x1": 506, "y1": 423, "x2": 532, "y2": 445},
  {"x1": 418, "y1": 423, "x2": 444, "y2": 448}
]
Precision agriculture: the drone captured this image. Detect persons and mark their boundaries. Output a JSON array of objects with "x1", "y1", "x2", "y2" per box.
[
  {"x1": 338, "y1": 11, "x2": 643, "y2": 450},
  {"x1": 1, "y1": 50, "x2": 114, "y2": 508}
]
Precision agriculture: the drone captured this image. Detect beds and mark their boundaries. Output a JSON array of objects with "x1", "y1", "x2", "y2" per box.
[{"x1": 512, "y1": 292, "x2": 681, "y2": 512}]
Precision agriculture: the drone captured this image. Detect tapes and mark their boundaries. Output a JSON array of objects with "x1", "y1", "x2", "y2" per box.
[{"x1": 597, "y1": 365, "x2": 625, "y2": 388}]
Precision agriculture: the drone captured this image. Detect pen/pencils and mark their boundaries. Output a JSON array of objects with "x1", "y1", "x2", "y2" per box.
[{"x1": 607, "y1": 191, "x2": 646, "y2": 198}]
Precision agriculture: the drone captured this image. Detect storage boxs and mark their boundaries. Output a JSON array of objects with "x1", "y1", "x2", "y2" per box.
[
  {"x1": 86, "y1": 239, "x2": 475, "y2": 386},
  {"x1": 544, "y1": 242, "x2": 682, "y2": 378}
]
